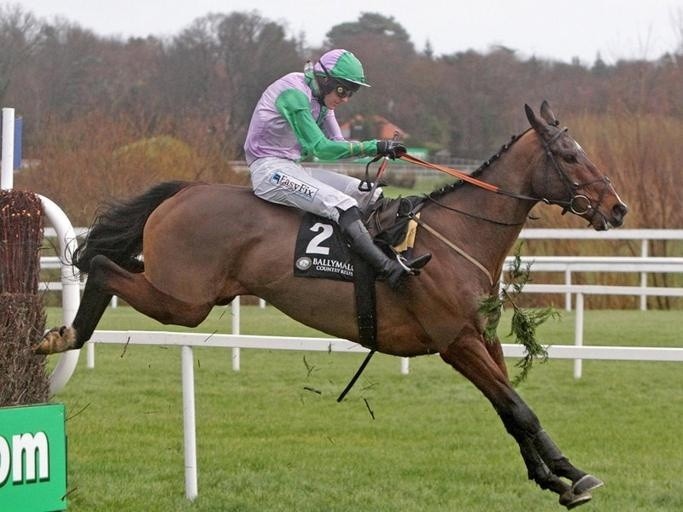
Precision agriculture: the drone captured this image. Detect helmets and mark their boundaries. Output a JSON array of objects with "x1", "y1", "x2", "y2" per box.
[{"x1": 311, "y1": 48, "x2": 372, "y2": 89}]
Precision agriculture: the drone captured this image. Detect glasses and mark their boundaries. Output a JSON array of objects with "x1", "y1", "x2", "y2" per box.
[{"x1": 326, "y1": 76, "x2": 354, "y2": 99}]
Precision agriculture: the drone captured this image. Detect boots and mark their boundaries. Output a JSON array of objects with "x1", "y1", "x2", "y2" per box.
[{"x1": 337, "y1": 205, "x2": 432, "y2": 296}]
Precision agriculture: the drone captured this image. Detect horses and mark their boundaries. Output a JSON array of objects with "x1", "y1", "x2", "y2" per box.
[{"x1": 31, "y1": 99, "x2": 629, "y2": 510}]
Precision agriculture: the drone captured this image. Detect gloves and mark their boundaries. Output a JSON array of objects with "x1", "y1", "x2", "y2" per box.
[{"x1": 376, "y1": 137, "x2": 408, "y2": 163}]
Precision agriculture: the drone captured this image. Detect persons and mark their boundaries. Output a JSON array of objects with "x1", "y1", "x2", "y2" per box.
[{"x1": 243, "y1": 48, "x2": 433, "y2": 292}]
